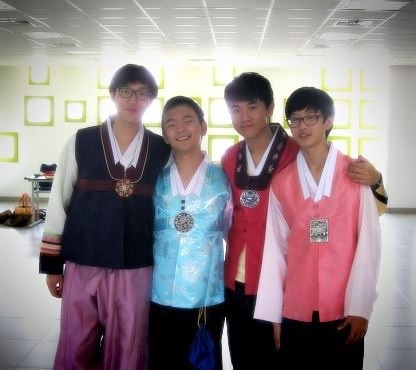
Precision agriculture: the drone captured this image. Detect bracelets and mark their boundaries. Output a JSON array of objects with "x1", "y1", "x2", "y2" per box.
[{"x1": 371, "y1": 172, "x2": 383, "y2": 190}]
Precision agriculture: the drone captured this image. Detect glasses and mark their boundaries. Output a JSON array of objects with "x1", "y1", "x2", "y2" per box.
[
  {"x1": 117, "y1": 87, "x2": 152, "y2": 100},
  {"x1": 285, "y1": 115, "x2": 324, "y2": 128}
]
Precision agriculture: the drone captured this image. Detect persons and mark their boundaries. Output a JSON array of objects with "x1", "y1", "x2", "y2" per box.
[
  {"x1": 39, "y1": 63, "x2": 172, "y2": 370},
  {"x1": 149, "y1": 96, "x2": 234, "y2": 370},
  {"x1": 253, "y1": 87, "x2": 381, "y2": 370},
  {"x1": 221, "y1": 72, "x2": 389, "y2": 370}
]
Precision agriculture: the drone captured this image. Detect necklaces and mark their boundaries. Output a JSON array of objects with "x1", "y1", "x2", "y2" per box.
[{"x1": 111, "y1": 121, "x2": 142, "y2": 197}]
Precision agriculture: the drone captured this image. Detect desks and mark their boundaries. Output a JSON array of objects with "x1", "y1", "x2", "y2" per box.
[{"x1": 24, "y1": 176, "x2": 52, "y2": 228}]
